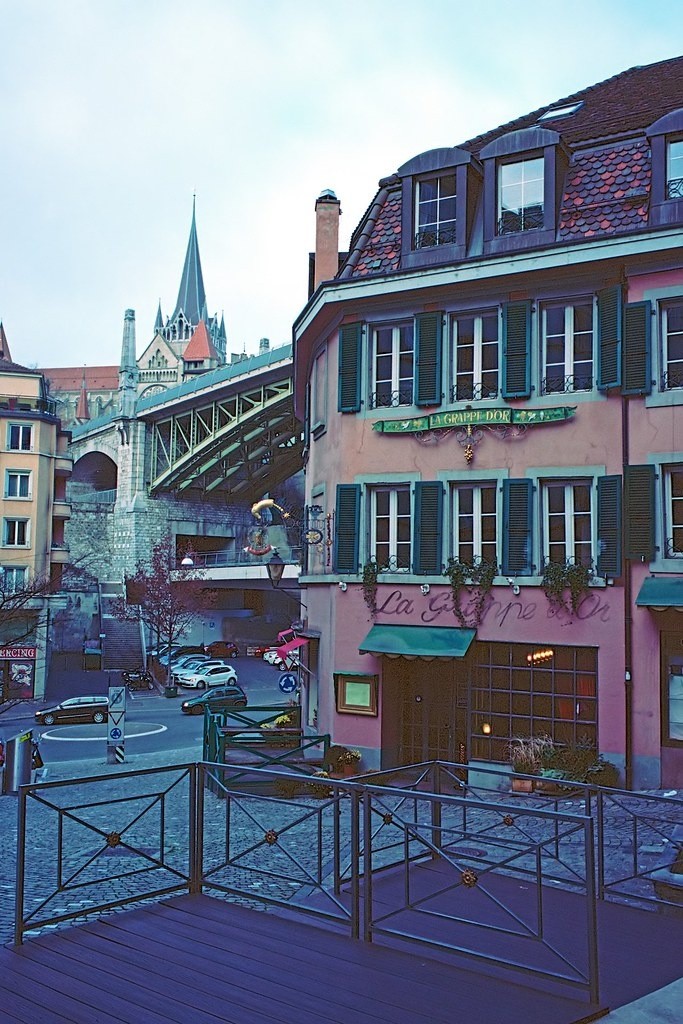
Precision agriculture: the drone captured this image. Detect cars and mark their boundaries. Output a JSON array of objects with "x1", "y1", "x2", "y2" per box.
[
  {"x1": 200, "y1": 641, "x2": 238, "y2": 659},
  {"x1": 181, "y1": 665, "x2": 238, "y2": 688},
  {"x1": 145, "y1": 640, "x2": 225, "y2": 688},
  {"x1": 259, "y1": 640, "x2": 299, "y2": 671},
  {"x1": 35, "y1": 695, "x2": 110, "y2": 725}
]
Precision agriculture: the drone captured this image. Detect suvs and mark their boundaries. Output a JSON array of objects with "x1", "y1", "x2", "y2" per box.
[{"x1": 179, "y1": 686, "x2": 248, "y2": 717}]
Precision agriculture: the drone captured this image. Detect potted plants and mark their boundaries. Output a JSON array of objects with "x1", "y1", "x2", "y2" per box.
[
  {"x1": 506, "y1": 731, "x2": 619, "y2": 796},
  {"x1": 306, "y1": 749, "x2": 386, "y2": 800},
  {"x1": 259, "y1": 714, "x2": 300, "y2": 749}
]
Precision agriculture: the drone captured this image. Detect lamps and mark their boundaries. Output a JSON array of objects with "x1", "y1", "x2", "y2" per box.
[{"x1": 264, "y1": 548, "x2": 286, "y2": 589}]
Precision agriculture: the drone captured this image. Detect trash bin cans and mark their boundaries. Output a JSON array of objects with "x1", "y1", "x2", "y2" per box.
[{"x1": 5, "y1": 727, "x2": 34, "y2": 796}]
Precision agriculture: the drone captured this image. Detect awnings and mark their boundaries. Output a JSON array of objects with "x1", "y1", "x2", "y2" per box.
[
  {"x1": 277, "y1": 628, "x2": 294, "y2": 643},
  {"x1": 634, "y1": 575, "x2": 683, "y2": 612},
  {"x1": 358, "y1": 623, "x2": 477, "y2": 662},
  {"x1": 276, "y1": 638, "x2": 310, "y2": 662}
]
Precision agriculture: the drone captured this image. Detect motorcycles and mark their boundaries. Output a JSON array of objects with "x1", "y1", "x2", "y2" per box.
[{"x1": 121, "y1": 666, "x2": 154, "y2": 691}]
